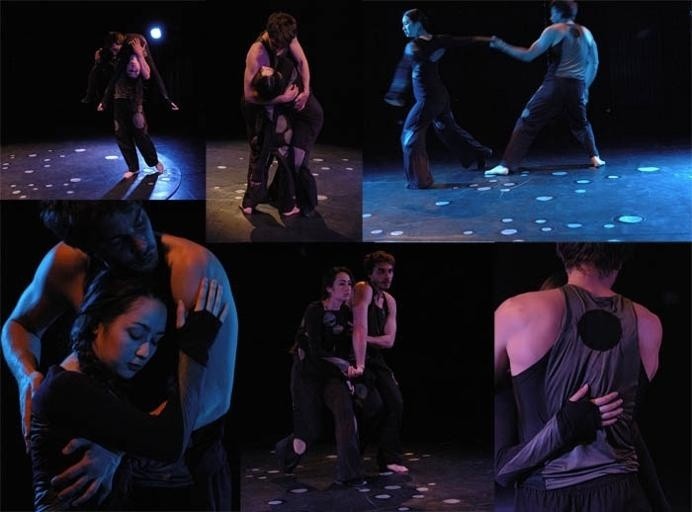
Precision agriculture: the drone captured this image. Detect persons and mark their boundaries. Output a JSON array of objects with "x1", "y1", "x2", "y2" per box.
[
  {"x1": 482, "y1": 0, "x2": 606, "y2": 178},
  {"x1": 252, "y1": 57, "x2": 323, "y2": 220},
  {"x1": 29, "y1": 262, "x2": 233, "y2": 512},
  {"x1": 93, "y1": 30, "x2": 167, "y2": 181},
  {"x1": 268, "y1": 264, "x2": 371, "y2": 481},
  {"x1": 0, "y1": 199, "x2": 239, "y2": 511},
  {"x1": 238, "y1": 8, "x2": 311, "y2": 216},
  {"x1": 81, "y1": 33, "x2": 181, "y2": 115},
  {"x1": 343, "y1": 250, "x2": 415, "y2": 473},
  {"x1": 383, "y1": 8, "x2": 504, "y2": 188}
]
